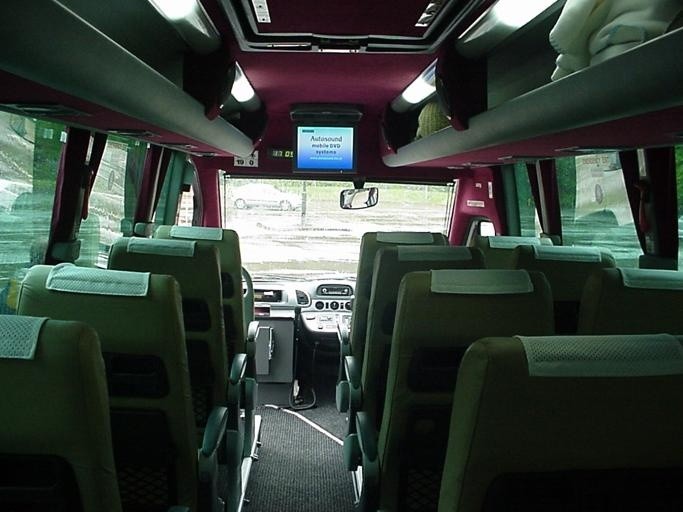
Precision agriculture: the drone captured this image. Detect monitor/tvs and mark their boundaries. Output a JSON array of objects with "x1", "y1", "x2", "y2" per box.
[{"x1": 293, "y1": 123, "x2": 359, "y2": 174}]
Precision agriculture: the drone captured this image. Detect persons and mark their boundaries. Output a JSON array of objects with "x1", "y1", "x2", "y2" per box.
[{"x1": 5, "y1": 236, "x2": 48, "y2": 311}]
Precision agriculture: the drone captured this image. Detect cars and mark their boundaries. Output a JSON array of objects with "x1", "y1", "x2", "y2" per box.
[
  {"x1": 233, "y1": 183, "x2": 302, "y2": 211},
  {"x1": 575, "y1": 154, "x2": 635, "y2": 228}
]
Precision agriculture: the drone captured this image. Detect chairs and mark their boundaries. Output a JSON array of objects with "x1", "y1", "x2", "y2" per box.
[{"x1": 0, "y1": 223, "x2": 259, "y2": 512}]
